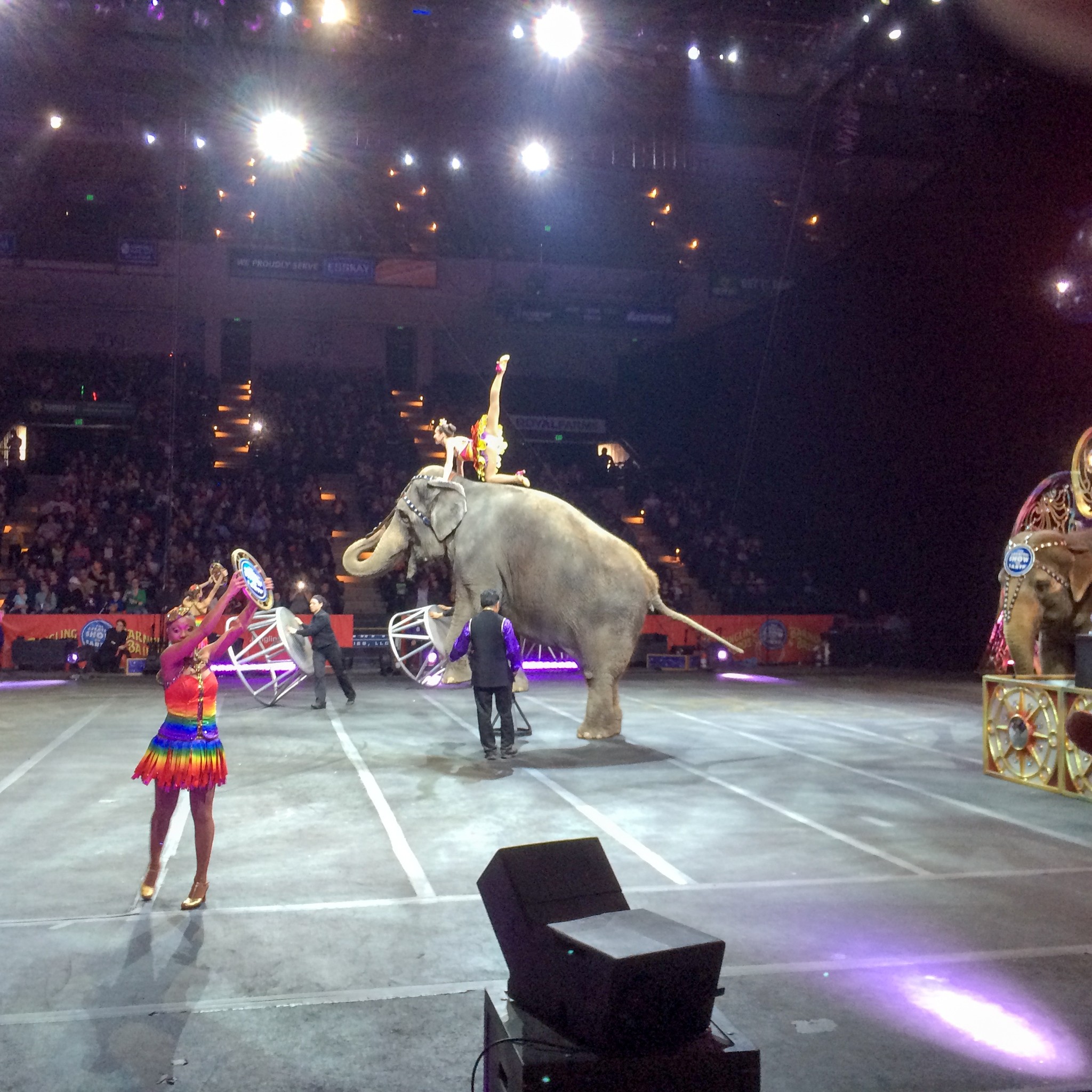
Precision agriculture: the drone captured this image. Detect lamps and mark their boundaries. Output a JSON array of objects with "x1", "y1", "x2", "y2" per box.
[{"x1": 68, "y1": 645, "x2": 96, "y2": 663}]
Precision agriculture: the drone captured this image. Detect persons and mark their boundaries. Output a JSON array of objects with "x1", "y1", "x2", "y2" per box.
[
  {"x1": 288, "y1": 595, "x2": 357, "y2": 710},
  {"x1": 95, "y1": 619, "x2": 131, "y2": 674},
  {"x1": 131, "y1": 571, "x2": 274, "y2": 910},
  {"x1": 182, "y1": 568, "x2": 228, "y2": 652},
  {"x1": 0, "y1": 344, "x2": 453, "y2": 614},
  {"x1": 449, "y1": 589, "x2": 524, "y2": 761},
  {"x1": 433, "y1": 355, "x2": 533, "y2": 489},
  {"x1": 416, "y1": 374, "x2": 918, "y2": 672},
  {"x1": 430, "y1": 596, "x2": 456, "y2": 620}
]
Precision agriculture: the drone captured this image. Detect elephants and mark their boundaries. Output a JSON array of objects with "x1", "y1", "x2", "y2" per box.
[
  {"x1": 342, "y1": 465, "x2": 743, "y2": 739},
  {"x1": 998, "y1": 528, "x2": 1092, "y2": 689}
]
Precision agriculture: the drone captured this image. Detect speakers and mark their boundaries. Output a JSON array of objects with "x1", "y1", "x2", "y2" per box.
[{"x1": 477, "y1": 837, "x2": 726, "y2": 1058}]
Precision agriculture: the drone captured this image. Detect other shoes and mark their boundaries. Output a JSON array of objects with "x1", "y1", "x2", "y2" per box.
[
  {"x1": 501, "y1": 748, "x2": 518, "y2": 758},
  {"x1": 485, "y1": 754, "x2": 496, "y2": 759},
  {"x1": 311, "y1": 701, "x2": 326, "y2": 709},
  {"x1": 346, "y1": 697, "x2": 354, "y2": 705}
]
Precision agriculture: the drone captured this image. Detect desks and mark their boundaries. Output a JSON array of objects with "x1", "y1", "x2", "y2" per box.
[
  {"x1": 389, "y1": 605, "x2": 450, "y2": 684},
  {"x1": 223, "y1": 607, "x2": 314, "y2": 705}
]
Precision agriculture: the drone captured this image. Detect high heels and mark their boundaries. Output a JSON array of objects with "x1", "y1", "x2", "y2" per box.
[
  {"x1": 515, "y1": 470, "x2": 530, "y2": 487},
  {"x1": 140, "y1": 868, "x2": 159, "y2": 900},
  {"x1": 495, "y1": 355, "x2": 510, "y2": 372},
  {"x1": 181, "y1": 882, "x2": 210, "y2": 910}
]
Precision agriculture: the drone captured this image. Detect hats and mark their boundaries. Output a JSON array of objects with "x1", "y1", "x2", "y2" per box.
[
  {"x1": 188, "y1": 583, "x2": 199, "y2": 593},
  {"x1": 312, "y1": 595, "x2": 327, "y2": 609},
  {"x1": 164, "y1": 607, "x2": 190, "y2": 625},
  {"x1": 69, "y1": 576, "x2": 81, "y2": 585}
]
